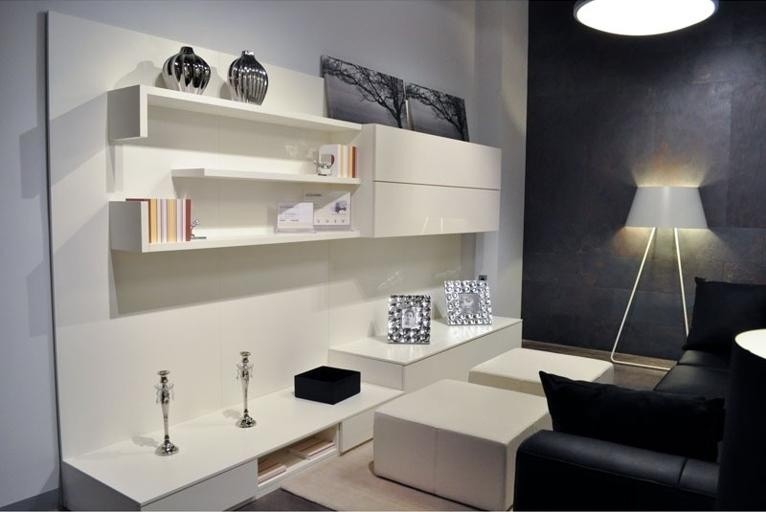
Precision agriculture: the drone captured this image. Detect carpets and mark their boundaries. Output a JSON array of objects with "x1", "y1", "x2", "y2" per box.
[{"x1": 280, "y1": 436, "x2": 514, "y2": 511}]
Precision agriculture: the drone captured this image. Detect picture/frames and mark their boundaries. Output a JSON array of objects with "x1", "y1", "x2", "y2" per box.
[
  {"x1": 386, "y1": 294, "x2": 431, "y2": 345},
  {"x1": 442, "y1": 279, "x2": 493, "y2": 326}
]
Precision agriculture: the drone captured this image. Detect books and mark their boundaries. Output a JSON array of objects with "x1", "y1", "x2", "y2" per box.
[
  {"x1": 321, "y1": 143, "x2": 356, "y2": 177},
  {"x1": 126, "y1": 197, "x2": 193, "y2": 245}
]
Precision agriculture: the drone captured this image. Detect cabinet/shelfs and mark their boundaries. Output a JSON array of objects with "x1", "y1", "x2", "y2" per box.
[{"x1": 44, "y1": 9, "x2": 523, "y2": 512}]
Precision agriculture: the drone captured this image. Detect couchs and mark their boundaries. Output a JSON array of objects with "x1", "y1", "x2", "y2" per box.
[{"x1": 513, "y1": 276, "x2": 766, "y2": 510}]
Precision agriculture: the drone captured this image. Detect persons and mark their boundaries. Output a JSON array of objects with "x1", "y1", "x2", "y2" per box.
[
  {"x1": 461, "y1": 296, "x2": 482, "y2": 313},
  {"x1": 405, "y1": 311, "x2": 414, "y2": 325}
]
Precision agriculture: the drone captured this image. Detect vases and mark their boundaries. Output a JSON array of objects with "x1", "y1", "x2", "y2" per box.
[
  {"x1": 227, "y1": 48, "x2": 269, "y2": 106},
  {"x1": 162, "y1": 45, "x2": 211, "y2": 94}
]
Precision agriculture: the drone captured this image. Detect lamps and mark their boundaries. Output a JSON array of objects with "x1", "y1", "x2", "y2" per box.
[
  {"x1": 574, "y1": 0, "x2": 718, "y2": 37},
  {"x1": 610, "y1": 184, "x2": 710, "y2": 371}
]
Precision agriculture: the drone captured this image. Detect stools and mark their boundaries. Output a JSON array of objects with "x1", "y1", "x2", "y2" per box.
[
  {"x1": 468, "y1": 346, "x2": 615, "y2": 398},
  {"x1": 373, "y1": 377, "x2": 553, "y2": 512}
]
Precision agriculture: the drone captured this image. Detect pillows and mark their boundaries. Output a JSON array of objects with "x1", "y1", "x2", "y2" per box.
[
  {"x1": 540, "y1": 370, "x2": 723, "y2": 464},
  {"x1": 683, "y1": 277, "x2": 766, "y2": 358}
]
